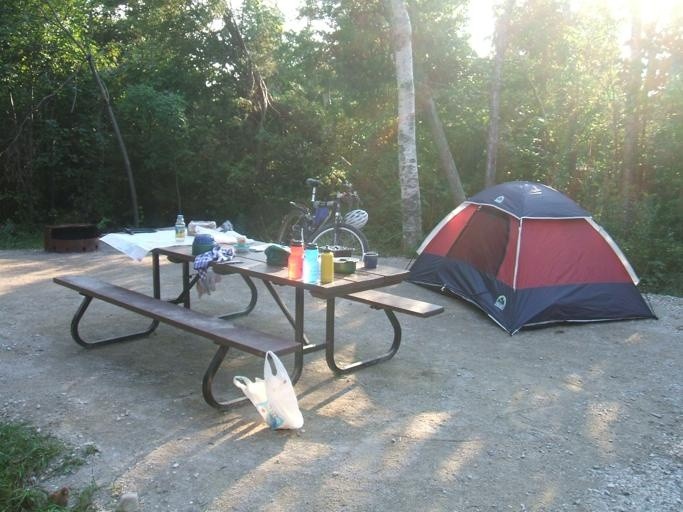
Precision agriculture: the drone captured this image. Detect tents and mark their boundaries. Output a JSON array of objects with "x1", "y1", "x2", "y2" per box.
[{"x1": 402, "y1": 179, "x2": 660, "y2": 337}]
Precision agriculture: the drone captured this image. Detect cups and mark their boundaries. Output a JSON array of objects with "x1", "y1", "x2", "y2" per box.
[{"x1": 363, "y1": 252, "x2": 379, "y2": 269}]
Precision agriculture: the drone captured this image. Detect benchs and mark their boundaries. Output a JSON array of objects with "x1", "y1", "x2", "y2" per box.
[
  {"x1": 51, "y1": 272, "x2": 303, "y2": 412},
  {"x1": 340, "y1": 287, "x2": 446, "y2": 375}
]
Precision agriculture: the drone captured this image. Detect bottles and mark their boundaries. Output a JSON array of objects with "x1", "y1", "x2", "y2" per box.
[
  {"x1": 174, "y1": 214, "x2": 186, "y2": 243},
  {"x1": 286, "y1": 238, "x2": 335, "y2": 285}
]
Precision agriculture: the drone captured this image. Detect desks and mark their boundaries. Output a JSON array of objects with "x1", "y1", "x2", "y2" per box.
[{"x1": 150, "y1": 241, "x2": 411, "y2": 374}]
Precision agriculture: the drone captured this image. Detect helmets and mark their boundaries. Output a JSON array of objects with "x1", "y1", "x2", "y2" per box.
[{"x1": 344, "y1": 209, "x2": 369, "y2": 231}]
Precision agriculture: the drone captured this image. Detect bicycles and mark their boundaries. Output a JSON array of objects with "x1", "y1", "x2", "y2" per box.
[{"x1": 274, "y1": 175, "x2": 370, "y2": 264}]
